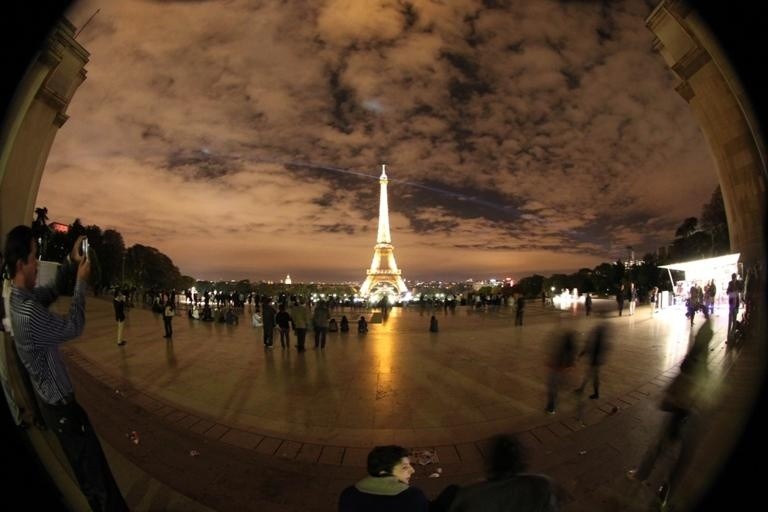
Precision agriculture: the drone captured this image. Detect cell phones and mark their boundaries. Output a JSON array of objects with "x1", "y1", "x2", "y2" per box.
[{"x1": 82, "y1": 237, "x2": 89, "y2": 261}]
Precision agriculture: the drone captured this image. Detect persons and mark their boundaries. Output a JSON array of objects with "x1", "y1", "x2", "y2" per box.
[
  {"x1": 262, "y1": 294, "x2": 387, "y2": 352},
  {"x1": 338, "y1": 432, "x2": 555, "y2": 512},
  {"x1": 586, "y1": 283, "x2": 658, "y2": 316},
  {"x1": 112, "y1": 285, "x2": 260, "y2": 345},
  {"x1": 419, "y1": 293, "x2": 514, "y2": 317},
  {"x1": 543, "y1": 331, "x2": 576, "y2": 415},
  {"x1": 627, "y1": 346, "x2": 709, "y2": 497},
  {"x1": 574, "y1": 325, "x2": 605, "y2": 398},
  {"x1": 686, "y1": 273, "x2": 740, "y2": 344},
  {"x1": 3, "y1": 225, "x2": 132, "y2": 512},
  {"x1": 515, "y1": 296, "x2": 525, "y2": 325},
  {"x1": 431, "y1": 316, "x2": 437, "y2": 332}
]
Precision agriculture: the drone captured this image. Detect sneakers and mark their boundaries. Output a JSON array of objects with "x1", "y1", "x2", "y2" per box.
[
  {"x1": 626, "y1": 468, "x2": 640, "y2": 480},
  {"x1": 544, "y1": 407, "x2": 555, "y2": 415},
  {"x1": 573, "y1": 388, "x2": 599, "y2": 400}
]
